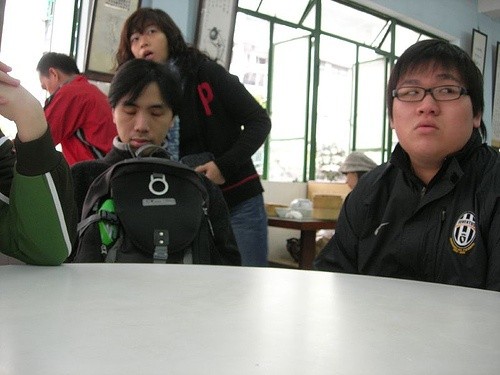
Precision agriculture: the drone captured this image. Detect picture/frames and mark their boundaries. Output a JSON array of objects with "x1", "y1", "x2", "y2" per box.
[
  {"x1": 84, "y1": 0, "x2": 142, "y2": 83},
  {"x1": 471, "y1": 28, "x2": 488, "y2": 77}
]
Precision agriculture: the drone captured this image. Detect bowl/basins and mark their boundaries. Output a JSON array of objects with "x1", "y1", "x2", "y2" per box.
[{"x1": 274, "y1": 206, "x2": 313, "y2": 218}]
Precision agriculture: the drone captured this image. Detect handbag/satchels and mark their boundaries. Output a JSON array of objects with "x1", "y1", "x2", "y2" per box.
[{"x1": 286, "y1": 238, "x2": 301, "y2": 263}]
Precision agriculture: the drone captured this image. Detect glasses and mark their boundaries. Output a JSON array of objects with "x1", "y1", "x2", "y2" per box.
[{"x1": 391, "y1": 85, "x2": 470, "y2": 102}]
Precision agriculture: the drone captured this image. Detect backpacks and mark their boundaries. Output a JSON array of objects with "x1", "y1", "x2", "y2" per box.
[{"x1": 74, "y1": 144, "x2": 242, "y2": 266}]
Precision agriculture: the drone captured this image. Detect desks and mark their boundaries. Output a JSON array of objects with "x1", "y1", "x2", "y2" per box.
[
  {"x1": 0, "y1": 264, "x2": 500, "y2": 375},
  {"x1": 268, "y1": 217, "x2": 339, "y2": 269}
]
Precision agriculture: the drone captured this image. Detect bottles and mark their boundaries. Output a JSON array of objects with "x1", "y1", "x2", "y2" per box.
[{"x1": 98, "y1": 199, "x2": 117, "y2": 245}]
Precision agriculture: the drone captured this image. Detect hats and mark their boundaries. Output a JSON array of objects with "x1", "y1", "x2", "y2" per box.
[{"x1": 341, "y1": 152, "x2": 377, "y2": 173}]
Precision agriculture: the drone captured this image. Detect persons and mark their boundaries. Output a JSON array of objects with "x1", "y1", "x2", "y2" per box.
[
  {"x1": 310, "y1": 39, "x2": 500, "y2": 295},
  {"x1": 117, "y1": 7, "x2": 272, "y2": 267},
  {"x1": 67, "y1": 58, "x2": 244, "y2": 267},
  {"x1": 37, "y1": 51, "x2": 117, "y2": 164},
  {"x1": 0, "y1": 63, "x2": 79, "y2": 269},
  {"x1": 339, "y1": 151, "x2": 378, "y2": 188}
]
